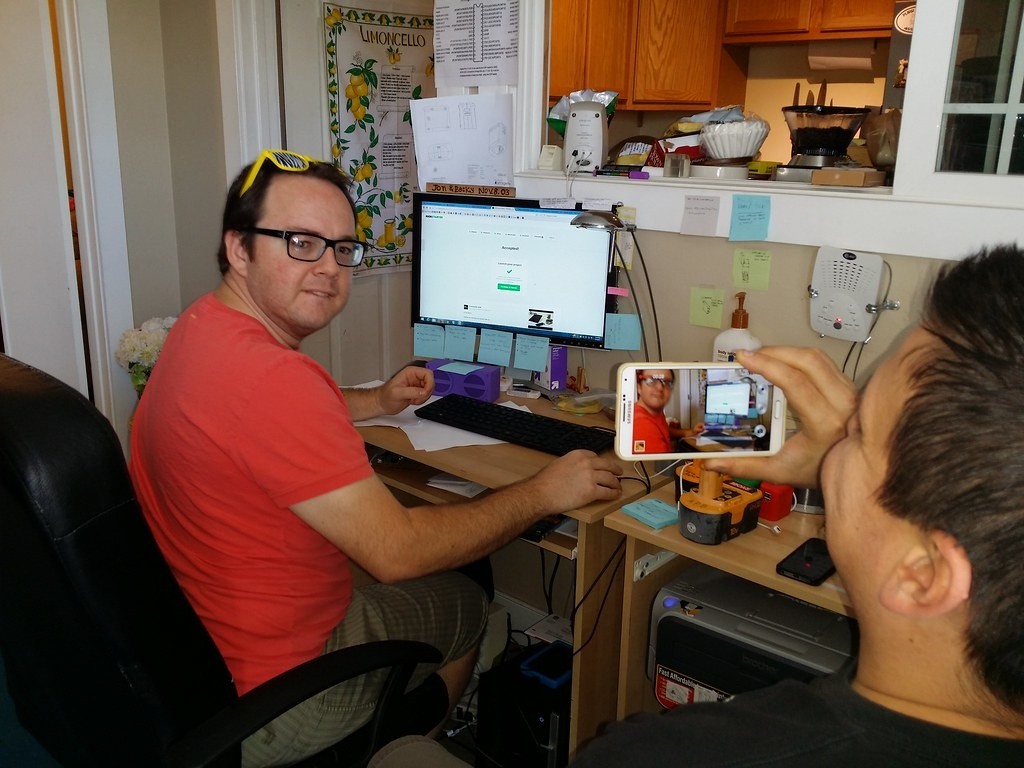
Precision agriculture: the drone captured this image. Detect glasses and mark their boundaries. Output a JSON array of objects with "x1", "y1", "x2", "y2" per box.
[
  {"x1": 638, "y1": 376, "x2": 675, "y2": 390},
  {"x1": 239, "y1": 149, "x2": 347, "y2": 198},
  {"x1": 242, "y1": 227, "x2": 368, "y2": 267}
]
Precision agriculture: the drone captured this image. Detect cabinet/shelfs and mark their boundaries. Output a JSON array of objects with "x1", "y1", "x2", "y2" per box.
[
  {"x1": 722, "y1": 0, "x2": 895, "y2": 45},
  {"x1": 548, "y1": 0, "x2": 748, "y2": 113},
  {"x1": 891, "y1": 0, "x2": 1023, "y2": 197}
]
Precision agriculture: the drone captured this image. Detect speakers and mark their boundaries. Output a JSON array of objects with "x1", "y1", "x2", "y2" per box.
[{"x1": 425, "y1": 360, "x2": 502, "y2": 404}]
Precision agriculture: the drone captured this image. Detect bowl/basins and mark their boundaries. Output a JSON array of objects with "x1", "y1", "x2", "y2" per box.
[
  {"x1": 783, "y1": 105, "x2": 869, "y2": 167},
  {"x1": 705, "y1": 122, "x2": 770, "y2": 157}
]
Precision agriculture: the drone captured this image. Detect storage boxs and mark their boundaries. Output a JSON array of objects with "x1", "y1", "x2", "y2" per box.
[{"x1": 812, "y1": 171, "x2": 886, "y2": 187}]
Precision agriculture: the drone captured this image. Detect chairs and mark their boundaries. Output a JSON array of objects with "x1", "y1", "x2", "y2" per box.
[{"x1": 0, "y1": 354, "x2": 453, "y2": 768}]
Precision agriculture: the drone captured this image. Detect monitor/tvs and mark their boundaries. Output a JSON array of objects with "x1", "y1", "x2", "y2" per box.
[
  {"x1": 705, "y1": 382, "x2": 750, "y2": 429},
  {"x1": 411, "y1": 192, "x2": 619, "y2": 402}
]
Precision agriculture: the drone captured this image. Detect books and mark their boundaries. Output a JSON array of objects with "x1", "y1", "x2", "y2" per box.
[
  {"x1": 518, "y1": 513, "x2": 578, "y2": 543},
  {"x1": 426, "y1": 472, "x2": 489, "y2": 498}
]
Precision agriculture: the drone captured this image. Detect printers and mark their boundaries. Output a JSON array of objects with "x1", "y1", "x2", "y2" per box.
[{"x1": 645, "y1": 562, "x2": 861, "y2": 712}]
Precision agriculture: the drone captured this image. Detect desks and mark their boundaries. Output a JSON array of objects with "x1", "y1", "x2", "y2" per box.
[{"x1": 327, "y1": 389, "x2": 852, "y2": 755}]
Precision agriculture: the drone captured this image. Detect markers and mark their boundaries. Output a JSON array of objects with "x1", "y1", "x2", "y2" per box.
[{"x1": 593, "y1": 170, "x2": 650, "y2": 179}]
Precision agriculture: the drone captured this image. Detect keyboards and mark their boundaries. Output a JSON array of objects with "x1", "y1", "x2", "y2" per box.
[
  {"x1": 702, "y1": 431, "x2": 753, "y2": 449},
  {"x1": 414, "y1": 393, "x2": 616, "y2": 457}
]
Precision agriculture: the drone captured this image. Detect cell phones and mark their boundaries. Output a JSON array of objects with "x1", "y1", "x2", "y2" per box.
[
  {"x1": 613, "y1": 362, "x2": 788, "y2": 462},
  {"x1": 521, "y1": 639, "x2": 575, "y2": 688},
  {"x1": 776, "y1": 538, "x2": 837, "y2": 586}
]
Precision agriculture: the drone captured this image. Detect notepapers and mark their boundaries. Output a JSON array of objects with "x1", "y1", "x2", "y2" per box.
[{"x1": 621, "y1": 499, "x2": 678, "y2": 530}]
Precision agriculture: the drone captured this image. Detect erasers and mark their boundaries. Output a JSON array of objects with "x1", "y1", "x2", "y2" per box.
[{"x1": 506, "y1": 388, "x2": 541, "y2": 399}]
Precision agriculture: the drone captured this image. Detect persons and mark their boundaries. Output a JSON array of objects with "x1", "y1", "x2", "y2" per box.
[
  {"x1": 632, "y1": 369, "x2": 705, "y2": 453},
  {"x1": 127, "y1": 147, "x2": 622, "y2": 768},
  {"x1": 569, "y1": 241, "x2": 1024, "y2": 768}
]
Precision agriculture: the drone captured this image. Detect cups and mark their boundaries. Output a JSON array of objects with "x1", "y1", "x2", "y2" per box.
[{"x1": 860, "y1": 105, "x2": 903, "y2": 169}]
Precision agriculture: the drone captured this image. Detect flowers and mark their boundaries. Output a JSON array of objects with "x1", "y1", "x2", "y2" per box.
[{"x1": 115, "y1": 314, "x2": 178, "y2": 399}]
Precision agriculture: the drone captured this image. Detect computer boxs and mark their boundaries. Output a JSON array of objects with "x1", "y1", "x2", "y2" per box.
[{"x1": 475, "y1": 639, "x2": 572, "y2": 768}]
